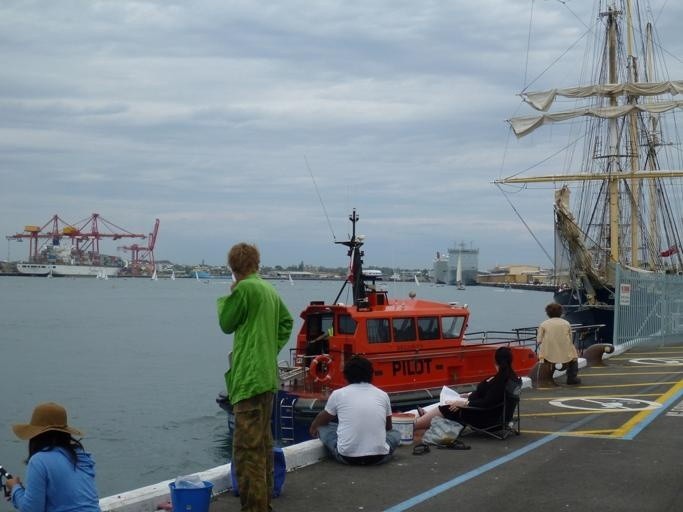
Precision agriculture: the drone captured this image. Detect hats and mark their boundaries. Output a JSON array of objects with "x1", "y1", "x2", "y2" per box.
[{"x1": 11, "y1": 401, "x2": 86, "y2": 441}]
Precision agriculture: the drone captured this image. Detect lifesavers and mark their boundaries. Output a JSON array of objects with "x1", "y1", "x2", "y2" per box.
[{"x1": 310, "y1": 355, "x2": 336, "y2": 385}]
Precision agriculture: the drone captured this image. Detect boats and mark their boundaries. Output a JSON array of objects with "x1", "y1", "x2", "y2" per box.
[{"x1": 216, "y1": 208, "x2": 539, "y2": 441}]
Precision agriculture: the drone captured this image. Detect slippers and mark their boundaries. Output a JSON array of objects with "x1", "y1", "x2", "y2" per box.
[
  {"x1": 437, "y1": 440, "x2": 471, "y2": 450},
  {"x1": 412, "y1": 443, "x2": 430, "y2": 457}
]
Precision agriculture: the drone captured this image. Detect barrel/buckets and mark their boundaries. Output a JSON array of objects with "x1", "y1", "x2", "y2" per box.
[
  {"x1": 169, "y1": 481, "x2": 215, "y2": 512},
  {"x1": 231, "y1": 449, "x2": 286, "y2": 499},
  {"x1": 391, "y1": 413, "x2": 415, "y2": 447}
]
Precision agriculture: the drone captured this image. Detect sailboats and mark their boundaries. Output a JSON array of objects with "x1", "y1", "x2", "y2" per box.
[
  {"x1": 457, "y1": 253, "x2": 465, "y2": 290},
  {"x1": 490, "y1": 1, "x2": 683, "y2": 349}
]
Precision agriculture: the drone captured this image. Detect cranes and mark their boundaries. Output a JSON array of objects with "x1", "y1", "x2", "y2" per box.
[{"x1": 5, "y1": 212, "x2": 159, "y2": 277}]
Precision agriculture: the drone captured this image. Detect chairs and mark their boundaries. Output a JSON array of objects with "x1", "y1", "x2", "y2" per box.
[
  {"x1": 453, "y1": 375, "x2": 523, "y2": 442},
  {"x1": 401, "y1": 318, "x2": 409, "y2": 332},
  {"x1": 447, "y1": 316, "x2": 458, "y2": 334},
  {"x1": 421, "y1": 318, "x2": 435, "y2": 338}
]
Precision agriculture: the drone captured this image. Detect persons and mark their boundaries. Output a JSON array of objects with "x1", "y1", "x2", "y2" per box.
[
  {"x1": 217, "y1": 243, "x2": 293, "y2": 512},
  {"x1": 413, "y1": 347, "x2": 522, "y2": 430},
  {"x1": 307, "y1": 356, "x2": 401, "y2": 465},
  {"x1": 535, "y1": 304, "x2": 581, "y2": 384},
  {"x1": 0, "y1": 404, "x2": 101, "y2": 511}
]
